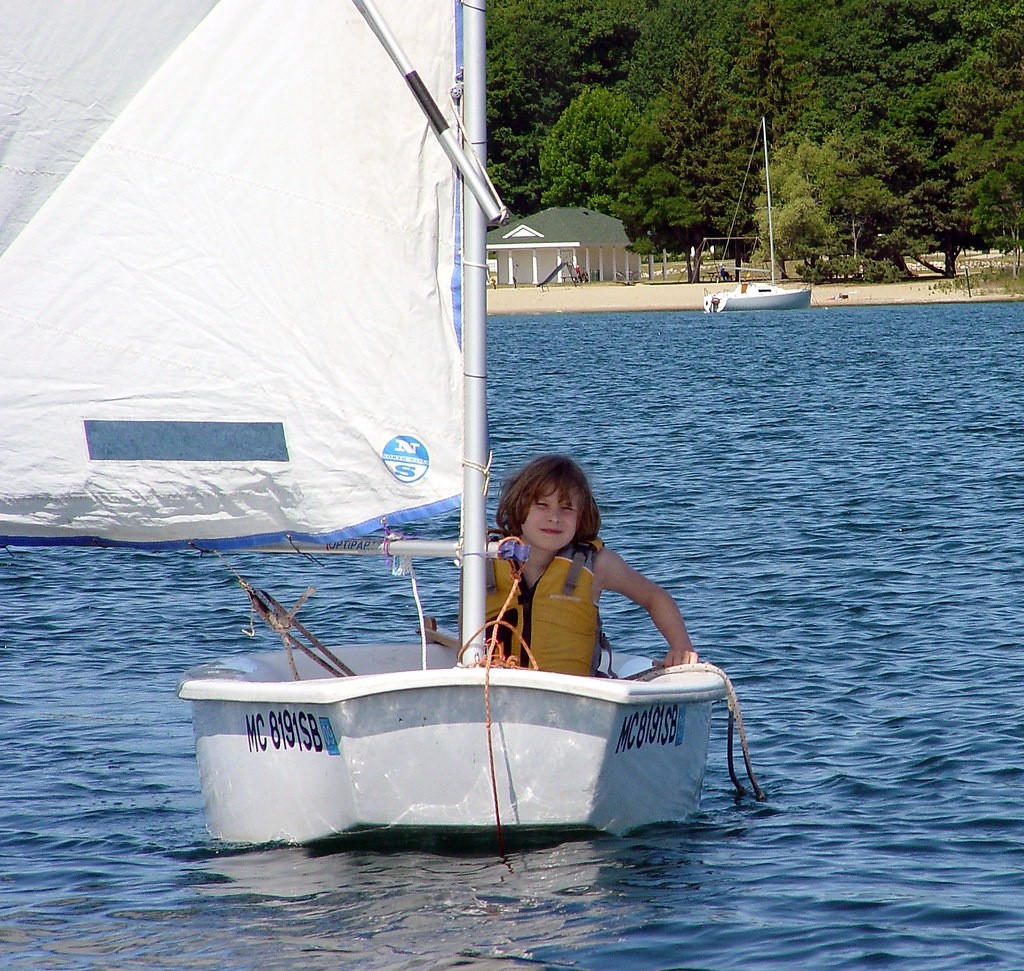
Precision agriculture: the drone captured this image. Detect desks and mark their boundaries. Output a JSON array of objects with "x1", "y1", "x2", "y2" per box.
[
  {"x1": 561, "y1": 276, "x2": 577, "y2": 283},
  {"x1": 706, "y1": 271, "x2": 721, "y2": 282}
]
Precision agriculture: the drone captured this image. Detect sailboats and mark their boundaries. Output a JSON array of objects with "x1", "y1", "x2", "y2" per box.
[
  {"x1": 0, "y1": 1, "x2": 727, "y2": 845},
  {"x1": 704, "y1": 117, "x2": 812, "y2": 313}
]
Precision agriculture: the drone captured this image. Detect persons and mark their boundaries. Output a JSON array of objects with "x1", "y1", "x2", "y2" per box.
[
  {"x1": 575, "y1": 265, "x2": 582, "y2": 283},
  {"x1": 721, "y1": 263, "x2": 729, "y2": 281},
  {"x1": 485, "y1": 453, "x2": 699, "y2": 677}
]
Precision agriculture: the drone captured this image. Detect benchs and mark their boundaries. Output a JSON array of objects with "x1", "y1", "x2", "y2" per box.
[{"x1": 701, "y1": 275, "x2": 733, "y2": 282}]
